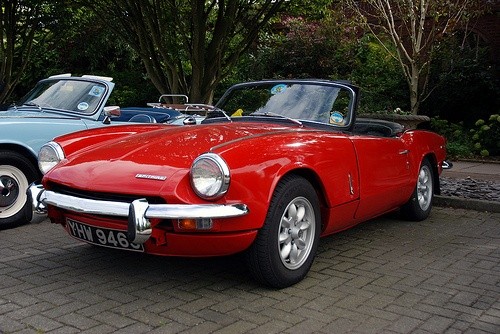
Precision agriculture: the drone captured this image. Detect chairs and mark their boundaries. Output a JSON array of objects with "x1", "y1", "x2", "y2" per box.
[{"x1": 128, "y1": 114, "x2": 157, "y2": 123}]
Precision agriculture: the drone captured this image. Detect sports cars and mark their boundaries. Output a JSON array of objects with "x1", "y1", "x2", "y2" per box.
[
  {"x1": 37, "y1": 79, "x2": 451, "y2": 290},
  {"x1": 0, "y1": 74, "x2": 211, "y2": 228}
]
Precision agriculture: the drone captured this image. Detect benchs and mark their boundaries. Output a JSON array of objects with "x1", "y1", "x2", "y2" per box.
[
  {"x1": 98, "y1": 110, "x2": 170, "y2": 123},
  {"x1": 354, "y1": 123, "x2": 392, "y2": 137}
]
